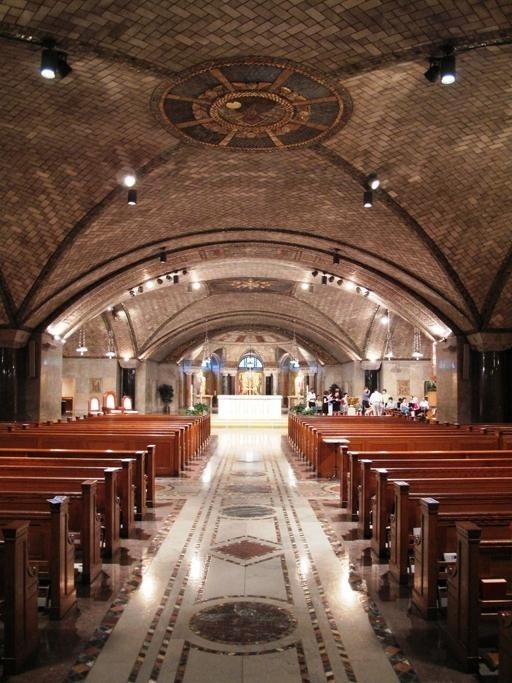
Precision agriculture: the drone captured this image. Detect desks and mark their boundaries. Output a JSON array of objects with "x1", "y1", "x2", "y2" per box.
[{"x1": 217, "y1": 394, "x2": 284, "y2": 419}]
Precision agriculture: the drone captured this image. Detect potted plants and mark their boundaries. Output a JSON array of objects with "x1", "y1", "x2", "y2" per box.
[{"x1": 159, "y1": 384, "x2": 173, "y2": 413}]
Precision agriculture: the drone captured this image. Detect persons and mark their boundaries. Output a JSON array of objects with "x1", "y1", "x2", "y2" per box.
[{"x1": 307, "y1": 386, "x2": 429, "y2": 415}]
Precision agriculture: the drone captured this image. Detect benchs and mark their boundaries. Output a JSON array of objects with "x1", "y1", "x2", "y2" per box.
[
  {"x1": 287, "y1": 408, "x2": 511, "y2": 683},
  {"x1": 0, "y1": 413, "x2": 212, "y2": 674}
]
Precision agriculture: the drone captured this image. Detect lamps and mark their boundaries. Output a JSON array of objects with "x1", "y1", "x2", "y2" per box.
[
  {"x1": 160, "y1": 252, "x2": 167, "y2": 264},
  {"x1": 422, "y1": 45, "x2": 456, "y2": 85},
  {"x1": 363, "y1": 174, "x2": 380, "y2": 208},
  {"x1": 201, "y1": 322, "x2": 210, "y2": 367},
  {"x1": 105, "y1": 330, "x2": 115, "y2": 360},
  {"x1": 332, "y1": 248, "x2": 340, "y2": 265},
  {"x1": 289, "y1": 320, "x2": 300, "y2": 368},
  {"x1": 40, "y1": 46, "x2": 72, "y2": 79},
  {"x1": 76, "y1": 330, "x2": 88, "y2": 355},
  {"x1": 124, "y1": 175, "x2": 137, "y2": 205},
  {"x1": 412, "y1": 327, "x2": 423, "y2": 360},
  {"x1": 384, "y1": 311, "x2": 394, "y2": 358},
  {"x1": 301, "y1": 282, "x2": 314, "y2": 295},
  {"x1": 310, "y1": 268, "x2": 373, "y2": 298},
  {"x1": 127, "y1": 267, "x2": 187, "y2": 297}
]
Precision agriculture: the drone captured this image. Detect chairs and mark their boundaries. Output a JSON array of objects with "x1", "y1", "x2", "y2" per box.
[{"x1": 88, "y1": 390, "x2": 138, "y2": 416}]
